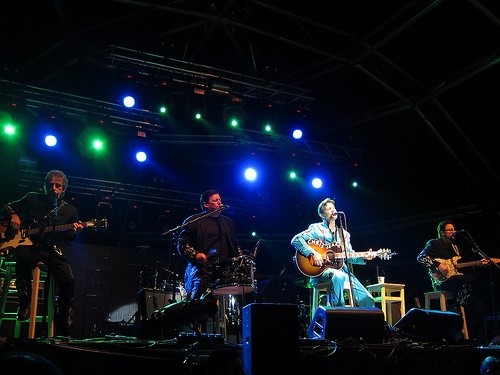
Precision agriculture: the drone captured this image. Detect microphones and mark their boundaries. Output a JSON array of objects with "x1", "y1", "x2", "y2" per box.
[
  {"x1": 452, "y1": 229, "x2": 465, "y2": 236},
  {"x1": 332, "y1": 212, "x2": 344, "y2": 215},
  {"x1": 219, "y1": 204, "x2": 230, "y2": 209}
]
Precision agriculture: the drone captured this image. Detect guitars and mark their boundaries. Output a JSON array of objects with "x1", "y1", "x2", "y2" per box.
[
  {"x1": 428, "y1": 255, "x2": 500, "y2": 284},
  {"x1": 295, "y1": 244, "x2": 392, "y2": 277},
  {"x1": 0, "y1": 218, "x2": 108, "y2": 249}
]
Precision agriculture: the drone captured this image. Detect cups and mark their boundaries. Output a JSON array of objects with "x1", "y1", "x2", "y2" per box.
[{"x1": 377, "y1": 277, "x2": 384, "y2": 284}]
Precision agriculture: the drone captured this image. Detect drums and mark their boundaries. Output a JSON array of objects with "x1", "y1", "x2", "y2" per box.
[
  {"x1": 209, "y1": 256, "x2": 257, "y2": 295},
  {"x1": 137, "y1": 264, "x2": 178, "y2": 300}
]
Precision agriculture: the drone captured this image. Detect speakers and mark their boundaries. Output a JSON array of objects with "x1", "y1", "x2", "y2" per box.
[
  {"x1": 309, "y1": 306, "x2": 385, "y2": 342},
  {"x1": 396, "y1": 308, "x2": 463, "y2": 340},
  {"x1": 485, "y1": 316, "x2": 500, "y2": 345},
  {"x1": 240, "y1": 303, "x2": 300, "y2": 375}
]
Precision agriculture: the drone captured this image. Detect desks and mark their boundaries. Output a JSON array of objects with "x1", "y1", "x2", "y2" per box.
[{"x1": 367, "y1": 284, "x2": 406, "y2": 324}]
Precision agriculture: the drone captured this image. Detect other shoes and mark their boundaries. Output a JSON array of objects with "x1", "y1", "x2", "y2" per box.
[
  {"x1": 16, "y1": 296, "x2": 32, "y2": 321},
  {"x1": 448, "y1": 306, "x2": 463, "y2": 316},
  {"x1": 55, "y1": 327, "x2": 71, "y2": 336}
]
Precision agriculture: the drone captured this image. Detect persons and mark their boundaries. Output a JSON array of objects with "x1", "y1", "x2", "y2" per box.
[
  {"x1": 416, "y1": 220, "x2": 490, "y2": 340},
  {"x1": 3, "y1": 170, "x2": 86, "y2": 339},
  {"x1": 291, "y1": 197, "x2": 383, "y2": 334},
  {"x1": 177, "y1": 188, "x2": 249, "y2": 336}
]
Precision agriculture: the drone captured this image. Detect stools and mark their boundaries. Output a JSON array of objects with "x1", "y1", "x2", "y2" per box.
[
  {"x1": 0, "y1": 261, "x2": 54, "y2": 339},
  {"x1": 206, "y1": 285, "x2": 227, "y2": 341},
  {"x1": 424, "y1": 291, "x2": 469, "y2": 340},
  {"x1": 311, "y1": 280, "x2": 354, "y2": 322}
]
